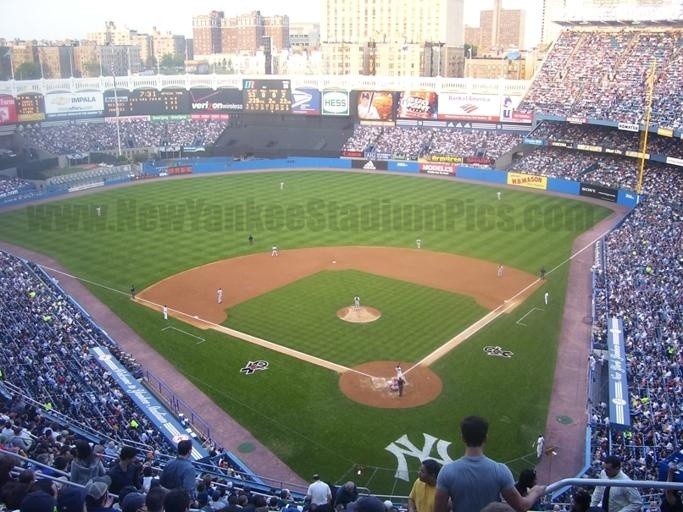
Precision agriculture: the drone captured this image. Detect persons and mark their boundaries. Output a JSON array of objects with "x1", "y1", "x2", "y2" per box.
[
  {"x1": 94, "y1": 205, "x2": 102, "y2": 217},
  {"x1": 214, "y1": 287, "x2": 224, "y2": 304},
  {"x1": 0, "y1": 26, "x2": 683, "y2": 198},
  {"x1": 543, "y1": 291, "x2": 549, "y2": 305},
  {"x1": 396, "y1": 376, "x2": 405, "y2": 398},
  {"x1": 540, "y1": 265, "x2": 546, "y2": 280},
  {"x1": 496, "y1": 263, "x2": 503, "y2": 277},
  {"x1": 585, "y1": 197, "x2": 683, "y2": 412},
  {"x1": 270, "y1": 244, "x2": 278, "y2": 256},
  {"x1": 496, "y1": 190, "x2": 502, "y2": 201},
  {"x1": 415, "y1": 237, "x2": 421, "y2": 249},
  {"x1": 0, "y1": 249, "x2": 147, "y2": 415},
  {"x1": 275, "y1": 246, "x2": 279, "y2": 255},
  {"x1": 351, "y1": 294, "x2": 360, "y2": 308},
  {"x1": 247, "y1": 233, "x2": 253, "y2": 246},
  {"x1": 394, "y1": 362, "x2": 410, "y2": 386},
  {"x1": 162, "y1": 304, "x2": 168, "y2": 320}
]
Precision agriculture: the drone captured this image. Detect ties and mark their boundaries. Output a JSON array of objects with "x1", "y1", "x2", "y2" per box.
[{"x1": 602, "y1": 486, "x2": 611, "y2": 511}]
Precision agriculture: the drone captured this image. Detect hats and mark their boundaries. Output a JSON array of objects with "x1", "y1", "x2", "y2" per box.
[
  {"x1": 20, "y1": 490, "x2": 58, "y2": 512},
  {"x1": 87, "y1": 475, "x2": 112, "y2": 500},
  {"x1": 59, "y1": 487, "x2": 85, "y2": 506}
]
